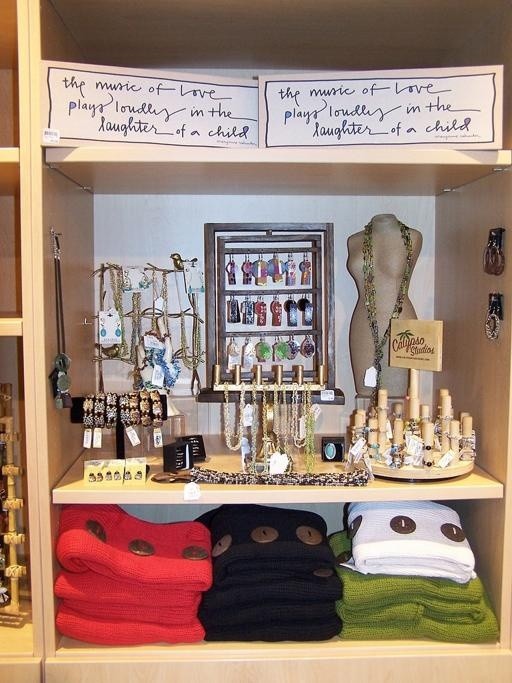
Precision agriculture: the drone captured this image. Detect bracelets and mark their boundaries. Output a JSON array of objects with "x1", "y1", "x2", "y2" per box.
[
  {"x1": 175, "y1": 437, "x2": 200, "y2": 470},
  {"x1": 350, "y1": 405, "x2": 477, "y2": 469},
  {"x1": 83, "y1": 390, "x2": 163, "y2": 428}
]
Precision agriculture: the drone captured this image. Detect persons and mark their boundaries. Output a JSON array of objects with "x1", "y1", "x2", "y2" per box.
[{"x1": 346, "y1": 214, "x2": 423, "y2": 398}]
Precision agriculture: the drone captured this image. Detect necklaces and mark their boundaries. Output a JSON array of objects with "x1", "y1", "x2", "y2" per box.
[
  {"x1": 222, "y1": 379, "x2": 313, "y2": 475},
  {"x1": 88, "y1": 259, "x2": 205, "y2": 391},
  {"x1": 363, "y1": 218, "x2": 413, "y2": 404}
]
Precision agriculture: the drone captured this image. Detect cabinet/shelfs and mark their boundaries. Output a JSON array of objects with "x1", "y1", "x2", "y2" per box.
[
  {"x1": 30, "y1": 0, "x2": 511, "y2": 680},
  {"x1": 0, "y1": 0, "x2": 43, "y2": 681}
]
